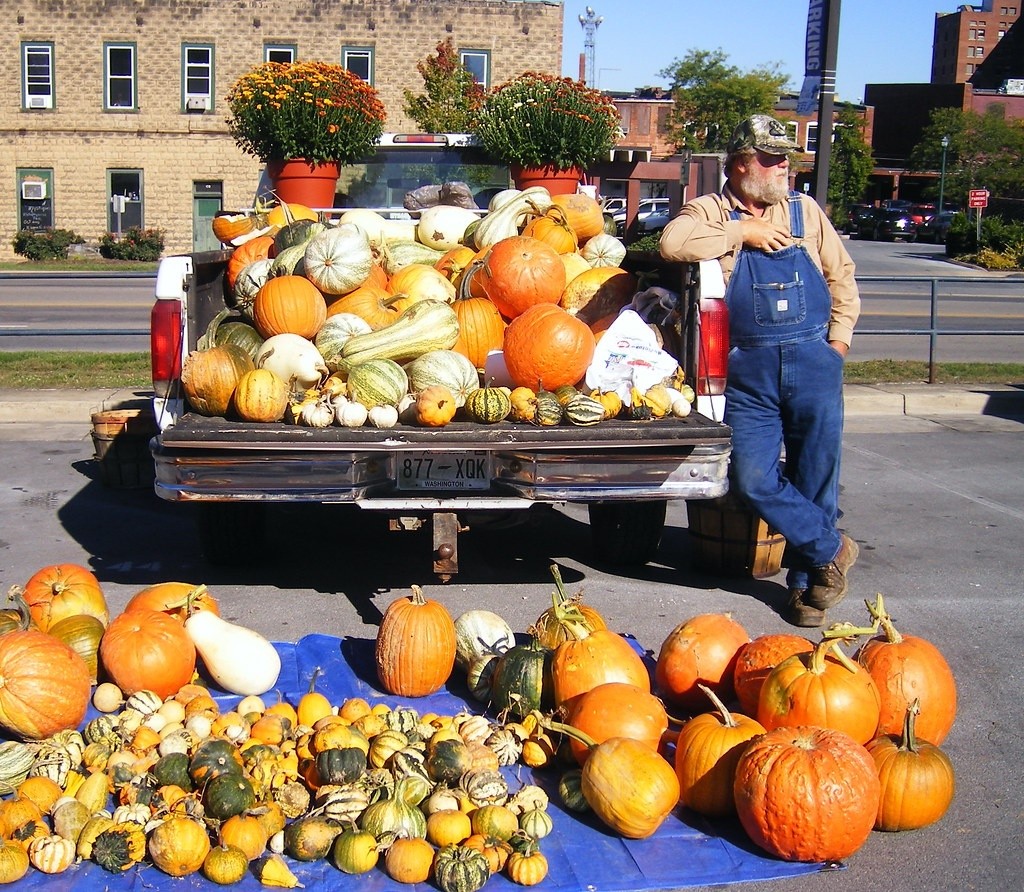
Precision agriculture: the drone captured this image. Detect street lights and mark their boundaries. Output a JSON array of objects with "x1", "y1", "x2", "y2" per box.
[{"x1": 938, "y1": 136, "x2": 949, "y2": 212}]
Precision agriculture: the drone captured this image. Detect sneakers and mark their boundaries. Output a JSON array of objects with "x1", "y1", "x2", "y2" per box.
[
  {"x1": 810, "y1": 531, "x2": 859, "y2": 609},
  {"x1": 787, "y1": 581, "x2": 826, "y2": 627}
]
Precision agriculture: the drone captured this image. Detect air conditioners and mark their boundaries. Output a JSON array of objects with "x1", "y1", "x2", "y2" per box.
[
  {"x1": 21, "y1": 181, "x2": 46, "y2": 200},
  {"x1": 187, "y1": 98, "x2": 206, "y2": 113},
  {"x1": 30, "y1": 97, "x2": 47, "y2": 109}
]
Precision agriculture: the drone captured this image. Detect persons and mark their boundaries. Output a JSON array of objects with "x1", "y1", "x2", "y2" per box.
[{"x1": 660, "y1": 111, "x2": 860, "y2": 626}]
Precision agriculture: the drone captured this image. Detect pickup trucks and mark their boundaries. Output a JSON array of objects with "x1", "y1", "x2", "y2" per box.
[{"x1": 148, "y1": 131, "x2": 732, "y2": 575}]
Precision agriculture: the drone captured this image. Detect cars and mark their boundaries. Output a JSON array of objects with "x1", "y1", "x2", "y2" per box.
[
  {"x1": 603, "y1": 198, "x2": 669, "y2": 236},
  {"x1": 834, "y1": 200, "x2": 976, "y2": 244}
]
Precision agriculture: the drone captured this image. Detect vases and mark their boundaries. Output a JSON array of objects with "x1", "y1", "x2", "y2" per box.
[
  {"x1": 266, "y1": 158, "x2": 342, "y2": 223},
  {"x1": 511, "y1": 160, "x2": 584, "y2": 196}
]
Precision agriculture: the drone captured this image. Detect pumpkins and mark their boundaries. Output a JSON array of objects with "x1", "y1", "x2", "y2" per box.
[
  {"x1": 181, "y1": 186, "x2": 695, "y2": 428},
  {"x1": 374, "y1": 563, "x2": 955, "y2": 862},
  {"x1": 0, "y1": 562, "x2": 555, "y2": 892}
]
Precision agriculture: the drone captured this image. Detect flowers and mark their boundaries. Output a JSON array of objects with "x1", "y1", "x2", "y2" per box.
[
  {"x1": 224, "y1": 59, "x2": 387, "y2": 159},
  {"x1": 472, "y1": 68, "x2": 623, "y2": 163}
]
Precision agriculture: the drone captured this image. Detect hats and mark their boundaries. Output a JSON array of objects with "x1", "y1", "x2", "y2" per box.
[{"x1": 725, "y1": 114, "x2": 806, "y2": 157}]
